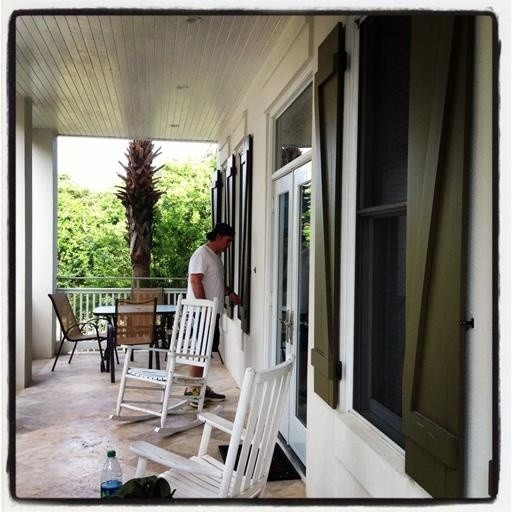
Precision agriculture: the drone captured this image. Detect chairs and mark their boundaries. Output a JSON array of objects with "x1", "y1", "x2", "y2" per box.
[
  {"x1": 48, "y1": 285, "x2": 224, "y2": 385},
  {"x1": 108, "y1": 292, "x2": 227, "y2": 435},
  {"x1": 128, "y1": 353, "x2": 297, "y2": 500}
]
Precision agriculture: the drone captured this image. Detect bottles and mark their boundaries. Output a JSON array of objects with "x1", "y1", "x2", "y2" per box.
[{"x1": 99, "y1": 449, "x2": 123, "y2": 499}]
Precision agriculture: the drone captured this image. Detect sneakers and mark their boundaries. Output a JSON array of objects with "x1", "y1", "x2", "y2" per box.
[{"x1": 185, "y1": 385, "x2": 226, "y2": 407}]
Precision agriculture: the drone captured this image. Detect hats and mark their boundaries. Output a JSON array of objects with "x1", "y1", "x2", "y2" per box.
[{"x1": 206, "y1": 222, "x2": 232, "y2": 236}]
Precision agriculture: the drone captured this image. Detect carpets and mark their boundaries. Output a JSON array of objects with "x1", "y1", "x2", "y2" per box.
[{"x1": 218, "y1": 442, "x2": 301, "y2": 481}]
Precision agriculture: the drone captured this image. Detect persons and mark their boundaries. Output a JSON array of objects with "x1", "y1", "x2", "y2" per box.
[{"x1": 178, "y1": 221, "x2": 242, "y2": 409}]
